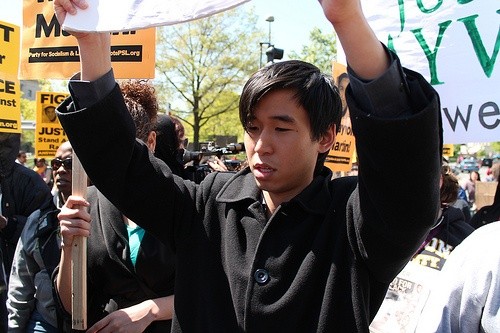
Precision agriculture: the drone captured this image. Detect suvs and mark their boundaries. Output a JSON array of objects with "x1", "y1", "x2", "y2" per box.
[{"x1": 481, "y1": 157, "x2": 492, "y2": 168}]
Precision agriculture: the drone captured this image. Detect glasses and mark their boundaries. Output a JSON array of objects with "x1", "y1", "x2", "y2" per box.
[{"x1": 51, "y1": 158, "x2": 72, "y2": 170}]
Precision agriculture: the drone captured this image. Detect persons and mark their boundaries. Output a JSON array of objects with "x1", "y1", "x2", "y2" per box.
[
  {"x1": 54, "y1": 1, "x2": 441, "y2": 333},
  {"x1": 368, "y1": 161, "x2": 500, "y2": 333},
  {"x1": 0, "y1": 80, "x2": 229, "y2": 333}
]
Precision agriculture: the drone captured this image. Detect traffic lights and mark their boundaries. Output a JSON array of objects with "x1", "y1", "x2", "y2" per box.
[{"x1": 266, "y1": 45, "x2": 284, "y2": 63}]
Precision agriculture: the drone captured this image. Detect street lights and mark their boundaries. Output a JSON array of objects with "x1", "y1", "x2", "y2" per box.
[{"x1": 265, "y1": 16, "x2": 274, "y2": 63}]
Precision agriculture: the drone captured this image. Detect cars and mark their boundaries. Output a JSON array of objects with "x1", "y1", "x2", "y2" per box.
[{"x1": 460, "y1": 160, "x2": 480, "y2": 173}]
